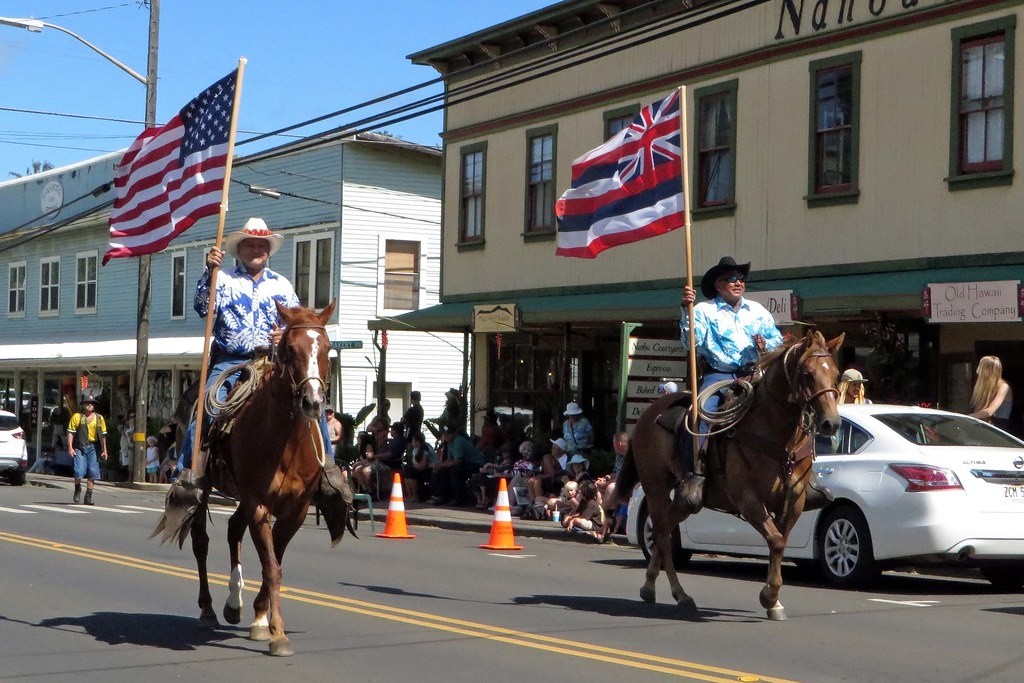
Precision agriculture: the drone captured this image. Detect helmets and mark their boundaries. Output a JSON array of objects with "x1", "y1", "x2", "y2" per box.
[{"x1": 442, "y1": 424, "x2": 456, "y2": 433}]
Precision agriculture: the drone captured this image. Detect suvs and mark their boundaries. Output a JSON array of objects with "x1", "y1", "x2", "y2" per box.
[{"x1": 0, "y1": 410, "x2": 28, "y2": 486}]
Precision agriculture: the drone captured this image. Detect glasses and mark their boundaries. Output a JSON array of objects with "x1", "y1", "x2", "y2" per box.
[
  {"x1": 718, "y1": 274, "x2": 746, "y2": 284},
  {"x1": 129, "y1": 416, "x2": 135, "y2": 418}
]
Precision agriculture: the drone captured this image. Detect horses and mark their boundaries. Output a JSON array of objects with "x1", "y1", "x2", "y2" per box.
[
  {"x1": 176, "y1": 297, "x2": 337, "y2": 657},
  {"x1": 609, "y1": 329, "x2": 846, "y2": 621}
]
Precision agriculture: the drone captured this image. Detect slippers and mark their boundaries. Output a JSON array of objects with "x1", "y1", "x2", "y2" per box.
[{"x1": 73, "y1": 484, "x2": 82, "y2": 503}]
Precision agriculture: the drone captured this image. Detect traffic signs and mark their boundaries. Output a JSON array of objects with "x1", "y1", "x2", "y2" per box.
[{"x1": 330, "y1": 341, "x2": 363, "y2": 349}]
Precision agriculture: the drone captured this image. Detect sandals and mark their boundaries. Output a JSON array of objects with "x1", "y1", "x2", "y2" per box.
[{"x1": 585, "y1": 530, "x2": 605, "y2": 543}]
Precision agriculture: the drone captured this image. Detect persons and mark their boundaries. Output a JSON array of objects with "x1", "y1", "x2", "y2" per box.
[
  {"x1": 678, "y1": 258, "x2": 783, "y2": 511},
  {"x1": 967, "y1": 356, "x2": 1013, "y2": 434},
  {"x1": 67, "y1": 393, "x2": 108, "y2": 506},
  {"x1": 836, "y1": 368, "x2": 873, "y2": 407},
  {"x1": 45, "y1": 389, "x2": 631, "y2": 541},
  {"x1": 174, "y1": 218, "x2": 353, "y2": 505},
  {"x1": 659, "y1": 381, "x2": 678, "y2": 398}
]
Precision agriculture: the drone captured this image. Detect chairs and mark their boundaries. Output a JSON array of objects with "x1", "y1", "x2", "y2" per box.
[{"x1": 315, "y1": 459, "x2": 375, "y2": 531}]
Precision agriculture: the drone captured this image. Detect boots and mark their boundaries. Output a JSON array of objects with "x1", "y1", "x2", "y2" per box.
[{"x1": 84, "y1": 490, "x2": 96, "y2": 505}]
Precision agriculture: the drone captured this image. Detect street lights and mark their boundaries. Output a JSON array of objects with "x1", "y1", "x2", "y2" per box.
[{"x1": 0, "y1": 17, "x2": 158, "y2": 481}]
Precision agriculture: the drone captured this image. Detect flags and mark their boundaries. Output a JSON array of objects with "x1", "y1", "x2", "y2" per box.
[
  {"x1": 102, "y1": 68, "x2": 239, "y2": 268},
  {"x1": 553, "y1": 85, "x2": 687, "y2": 259}
]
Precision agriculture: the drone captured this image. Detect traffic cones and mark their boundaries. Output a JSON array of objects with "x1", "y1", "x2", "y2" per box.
[
  {"x1": 478, "y1": 478, "x2": 525, "y2": 551},
  {"x1": 375, "y1": 473, "x2": 417, "y2": 538}
]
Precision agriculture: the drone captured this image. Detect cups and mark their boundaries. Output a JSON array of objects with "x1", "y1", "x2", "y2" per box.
[{"x1": 552, "y1": 511, "x2": 560, "y2": 522}]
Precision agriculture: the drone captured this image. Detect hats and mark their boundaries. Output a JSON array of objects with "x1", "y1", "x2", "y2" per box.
[
  {"x1": 659, "y1": 381, "x2": 678, "y2": 395},
  {"x1": 325, "y1": 404, "x2": 336, "y2": 412},
  {"x1": 147, "y1": 436, "x2": 158, "y2": 444},
  {"x1": 841, "y1": 368, "x2": 869, "y2": 383},
  {"x1": 388, "y1": 422, "x2": 406, "y2": 431},
  {"x1": 80, "y1": 395, "x2": 99, "y2": 405},
  {"x1": 550, "y1": 437, "x2": 567, "y2": 452},
  {"x1": 701, "y1": 256, "x2": 752, "y2": 300},
  {"x1": 567, "y1": 454, "x2": 590, "y2": 473},
  {"x1": 563, "y1": 402, "x2": 583, "y2": 415},
  {"x1": 224, "y1": 217, "x2": 284, "y2": 261},
  {"x1": 444, "y1": 388, "x2": 459, "y2": 399}
]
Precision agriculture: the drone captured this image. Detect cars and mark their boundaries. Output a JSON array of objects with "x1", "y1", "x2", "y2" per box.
[{"x1": 625, "y1": 403, "x2": 1024, "y2": 593}]
